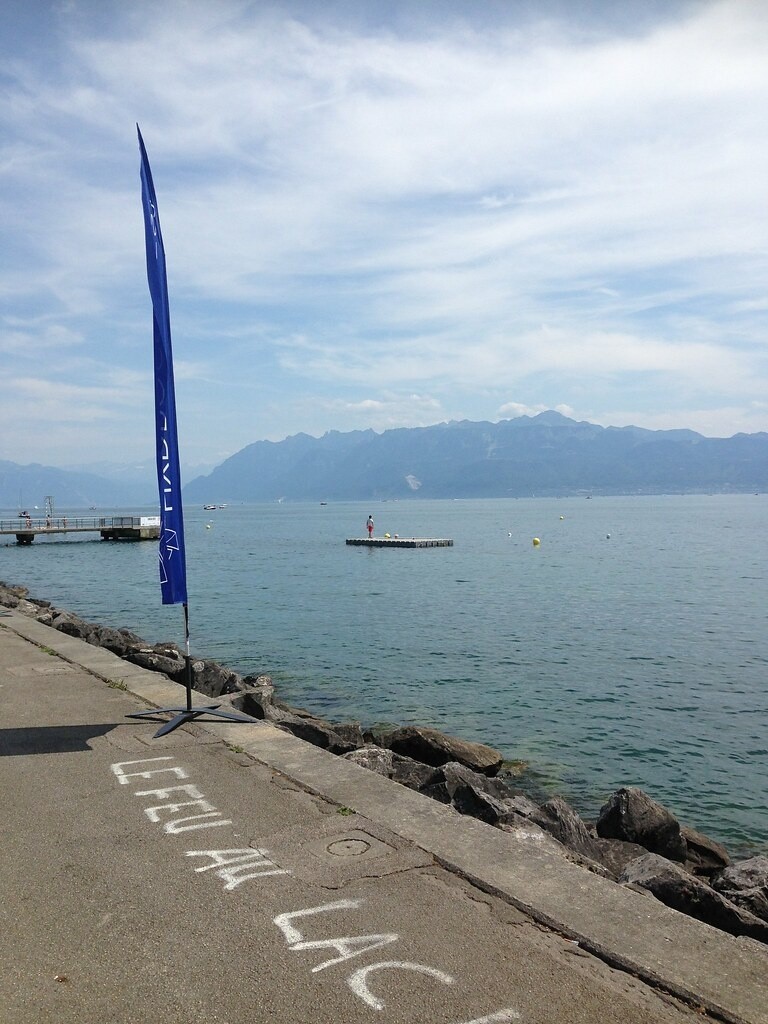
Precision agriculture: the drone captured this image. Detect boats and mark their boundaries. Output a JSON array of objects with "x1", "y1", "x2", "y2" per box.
[
  {"x1": 662, "y1": 494, "x2": 667, "y2": 496},
  {"x1": 320, "y1": 501, "x2": 328, "y2": 507},
  {"x1": 219, "y1": 503, "x2": 228, "y2": 510},
  {"x1": 586, "y1": 496, "x2": 593, "y2": 500},
  {"x1": 394, "y1": 497, "x2": 398, "y2": 501},
  {"x1": 203, "y1": 504, "x2": 217, "y2": 510},
  {"x1": 31, "y1": 506, "x2": 40, "y2": 510},
  {"x1": 453, "y1": 497, "x2": 460, "y2": 501},
  {"x1": 89, "y1": 506, "x2": 97, "y2": 510},
  {"x1": 18, "y1": 511, "x2": 30, "y2": 519},
  {"x1": 555, "y1": 495, "x2": 560, "y2": 501},
  {"x1": 381, "y1": 500, "x2": 387, "y2": 502},
  {"x1": 754, "y1": 493, "x2": 758, "y2": 495}
]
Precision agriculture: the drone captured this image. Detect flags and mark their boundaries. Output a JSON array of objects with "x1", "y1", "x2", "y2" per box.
[{"x1": 125, "y1": 122, "x2": 256, "y2": 737}]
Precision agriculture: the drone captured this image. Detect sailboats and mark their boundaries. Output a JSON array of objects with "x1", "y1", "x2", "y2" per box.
[{"x1": 531, "y1": 492, "x2": 535, "y2": 498}]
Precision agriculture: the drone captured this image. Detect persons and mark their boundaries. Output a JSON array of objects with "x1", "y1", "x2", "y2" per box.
[
  {"x1": 46, "y1": 514, "x2": 51, "y2": 529},
  {"x1": 366, "y1": 515, "x2": 374, "y2": 538},
  {"x1": 62, "y1": 516, "x2": 67, "y2": 528},
  {"x1": 26, "y1": 516, "x2": 30, "y2": 530}
]
